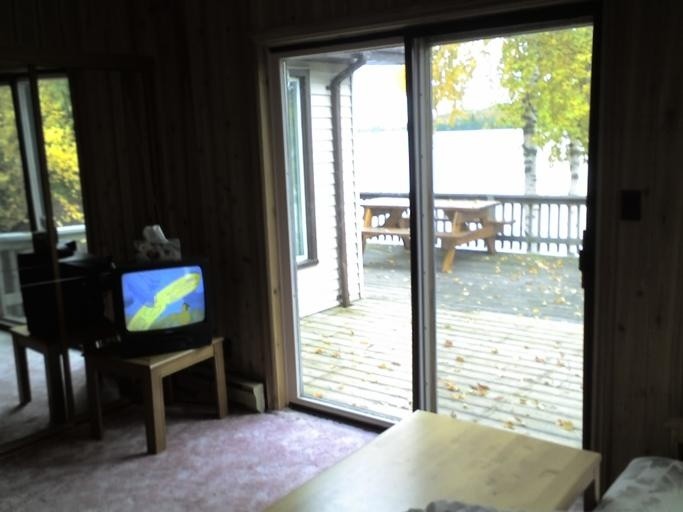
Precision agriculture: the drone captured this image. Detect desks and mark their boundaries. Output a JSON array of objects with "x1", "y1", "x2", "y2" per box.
[
  {"x1": 80, "y1": 330, "x2": 230, "y2": 457},
  {"x1": 361, "y1": 195, "x2": 502, "y2": 273},
  {"x1": 263, "y1": 405, "x2": 601, "y2": 512},
  {"x1": 7, "y1": 318, "x2": 113, "y2": 440}
]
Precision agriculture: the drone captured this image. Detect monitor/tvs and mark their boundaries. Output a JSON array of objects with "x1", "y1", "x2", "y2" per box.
[
  {"x1": 110, "y1": 259, "x2": 213, "y2": 355},
  {"x1": 18, "y1": 246, "x2": 110, "y2": 341}
]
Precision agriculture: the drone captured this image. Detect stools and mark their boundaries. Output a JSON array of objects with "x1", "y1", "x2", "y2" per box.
[{"x1": 597, "y1": 453, "x2": 681, "y2": 512}]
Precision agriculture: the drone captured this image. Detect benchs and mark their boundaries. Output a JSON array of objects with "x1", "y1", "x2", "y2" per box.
[
  {"x1": 360, "y1": 225, "x2": 470, "y2": 275},
  {"x1": 431, "y1": 216, "x2": 515, "y2": 255}
]
